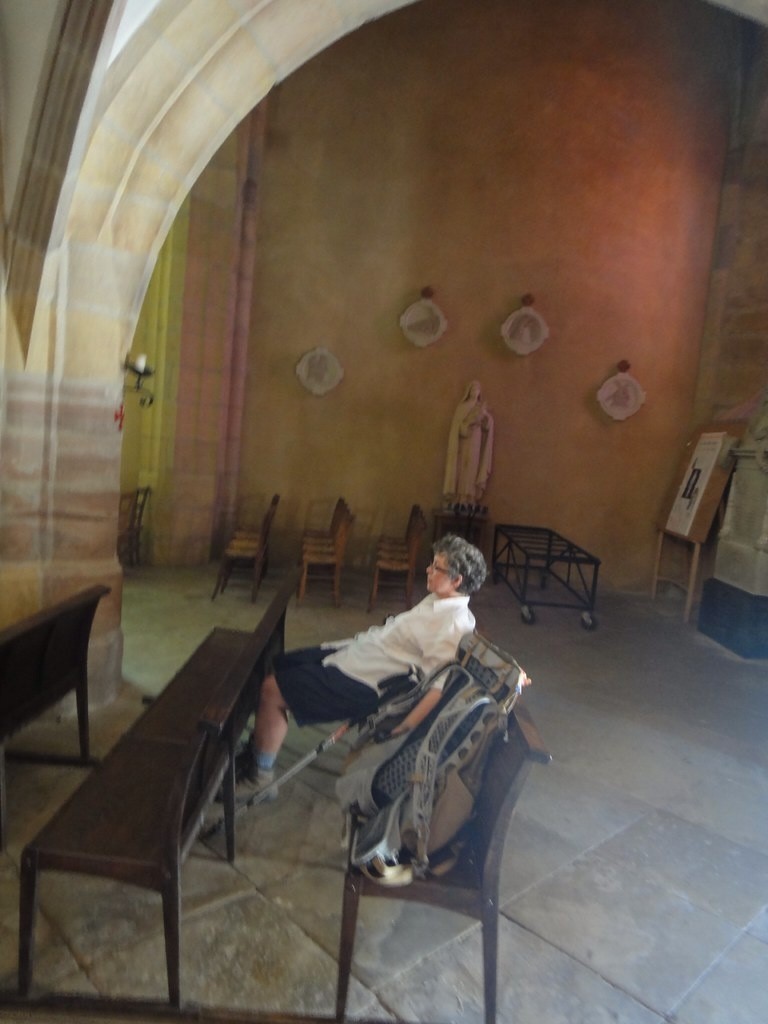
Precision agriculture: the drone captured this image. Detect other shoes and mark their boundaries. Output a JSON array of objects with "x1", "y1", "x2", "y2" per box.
[
  {"x1": 214, "y1": 765, "x2": 277, "y2": 802},
  {"x1": 225, "y1": 748, "x2": 250, "y2": 777}
]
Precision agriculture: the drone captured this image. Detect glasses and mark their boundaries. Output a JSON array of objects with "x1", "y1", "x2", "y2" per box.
[{"x1": 430, "y1": 562, "x2": 453, "y2": 575}]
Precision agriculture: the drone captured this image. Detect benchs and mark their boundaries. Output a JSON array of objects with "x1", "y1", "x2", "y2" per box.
[
  {"x1": 117, "y1": 486, "x2": 150, "y2": 574},
  {"x1": 15, "y1": 581, "x2": 299, "y2": 1011},
  {"x1": 0, "y1": 584, "x2": 115, "y2": 850},
  {"x1": 336, "y1": 692, "x2": 551, "y2": 1024}
]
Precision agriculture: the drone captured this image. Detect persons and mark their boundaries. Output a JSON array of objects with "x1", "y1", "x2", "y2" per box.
[
  {"x1": 212, "y1": 535, "x2": 487, "y2": 803},
  {"x1": 442, "y1": 380, "x2": 494, "y2": 504}
]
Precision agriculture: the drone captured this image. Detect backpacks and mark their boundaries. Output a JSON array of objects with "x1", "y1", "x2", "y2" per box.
[{"x1": 336, "y1": 629, "x2": 529, "y2": 886}]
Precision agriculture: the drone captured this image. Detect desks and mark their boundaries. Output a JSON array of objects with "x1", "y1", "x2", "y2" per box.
[{"x1": 434, "y1": 510, "x2": 485, "y2": 550}]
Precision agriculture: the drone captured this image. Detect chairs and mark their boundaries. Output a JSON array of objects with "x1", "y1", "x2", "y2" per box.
[
  {"x1": 298, "y1": 497, "x2": 356, "y2": 607},
  {"x1": 211, "y1": 493, "x2": 281, "y2": 603},
  {"x1": 369, "y1": 502, "x2": 426, "y2": 614}
]
